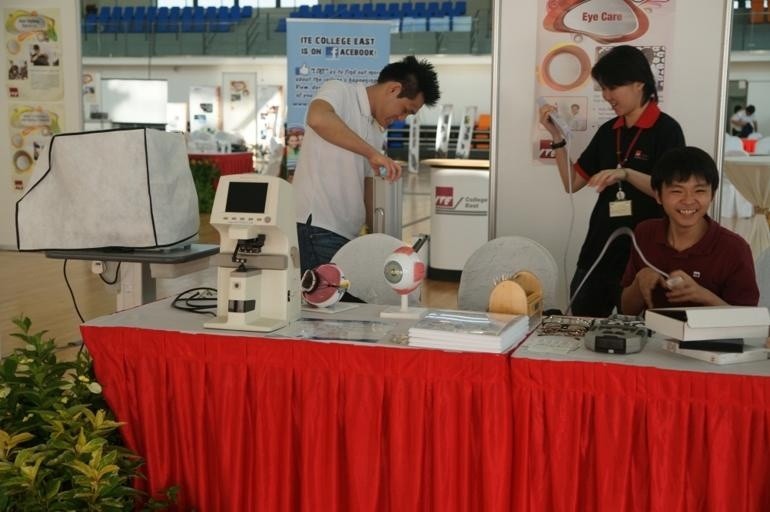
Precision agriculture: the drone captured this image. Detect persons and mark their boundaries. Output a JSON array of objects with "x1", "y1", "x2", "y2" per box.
[
  {"x1": 260, "y1": 105, "x2": 279, "y2": 153},
  {"x1": 281, "y1": 53, "x2": 443, "y2": 308},
  {"x1": 565, "y1": 103, "x2": 587, "y2": 130},
  {"x1": 286, "y1": 125, "x2": 305, "y2": 184},
  {"x1": 228, "y1": 79, "x2": 249, "y2": 98},
  {"x1": 7, "y1": 58, "x2": 19, "y2": 80},
  {"x1": 728, "y1": 103, "x2": 759, "y2": 137},
  {"x1": 30, "y1": 43, "x2": 51, "y2": 67},
  {"x1": 619, "y1": 146, "x2": 762, "y2": 317},
  {"x1": 728, "y1": 105, "x2": 744, "y2": 136},
  {"x1": 537, "y1": 46, "x2": 688, "y2": 318}
]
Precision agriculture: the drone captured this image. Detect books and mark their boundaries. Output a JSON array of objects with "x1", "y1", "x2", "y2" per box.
[
  {"x1": 675, "y1": 339, "x2": 748, "y2": 356},
  {"x1": 528, "y1": 314, "x2": 591, "y2": 355},
  {"x1": 380, "y1": 307, "x2": 531, "y2": 354},
  {"x1": 641, "y1": 302, "x2": 770, "y2": 343},
  {"x1": 660, "y1": 337, "x2": 770, "y2": 367}
]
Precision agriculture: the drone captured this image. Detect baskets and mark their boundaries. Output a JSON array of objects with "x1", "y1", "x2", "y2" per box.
[{"x1": 188, "y1": 152, "x2": 254, "y2": 195}]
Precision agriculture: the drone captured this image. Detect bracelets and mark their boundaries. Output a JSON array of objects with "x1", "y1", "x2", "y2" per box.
[
  {"x1": 623, "y1": 167, "x2": 629, "y2": 183},
  {"x1": 547, "y1": 137, "x2": 568, "y2": 149}
]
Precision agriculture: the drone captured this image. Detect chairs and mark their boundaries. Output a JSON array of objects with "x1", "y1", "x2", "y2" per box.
[
  {"x1": 755, "y1": 246, "x2": 770, "y2": 308},
  {"x1": 328, "y1": 234, "x2": 561, "y2": 315}
]
